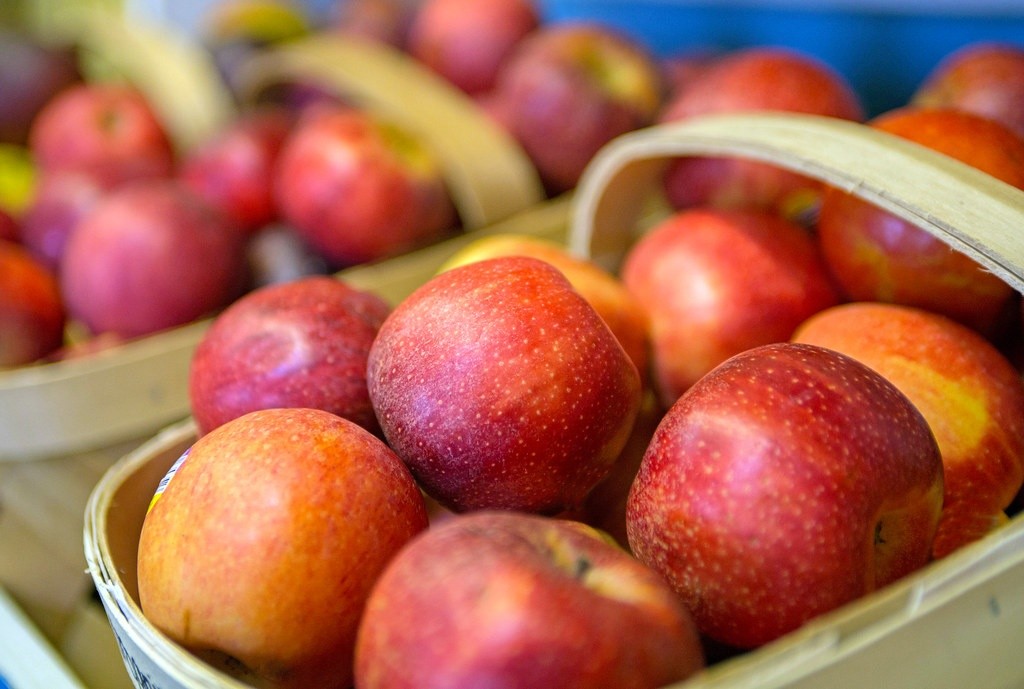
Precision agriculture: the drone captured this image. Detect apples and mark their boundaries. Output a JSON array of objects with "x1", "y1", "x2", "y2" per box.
[{"x1": 0, "y1": 0, "x2": 1024, "y2": 689}]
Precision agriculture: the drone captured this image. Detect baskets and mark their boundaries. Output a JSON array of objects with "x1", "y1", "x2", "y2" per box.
[{"x1": 0, "y1": 0, "x2": 1021, "y2": 689}]
